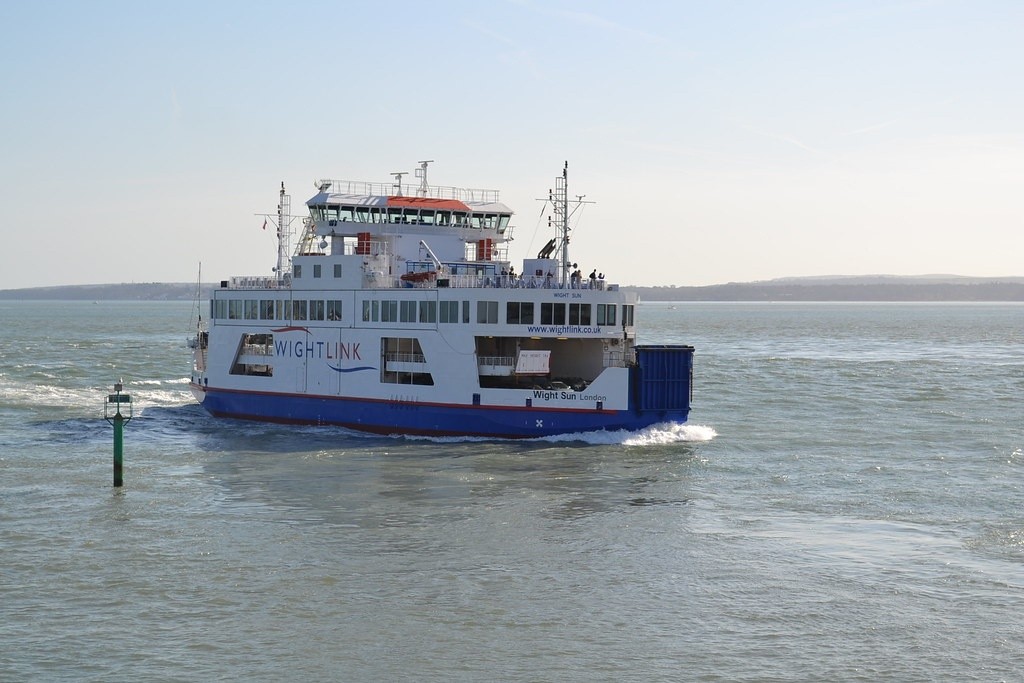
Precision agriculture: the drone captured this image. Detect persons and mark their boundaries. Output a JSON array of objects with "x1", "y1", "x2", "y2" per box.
[
  {"x1": 547, "y1": 270, "x2": 553, "y2": 286},
  {"x1": 509, "y1": 267, "x2": 514, "y2": 283},
  {"x1": 501, "y1": 267, "x2": 508, "y2": 278},
  {"x1": 571, "y1": 270, "x2": 582, "y2": 289},
  {"x1": 598, "y1": 273, "x2": 604, "y2": 279},
  {"x1": 589, "y1": 269, "x2": 596, "y2": 289}
]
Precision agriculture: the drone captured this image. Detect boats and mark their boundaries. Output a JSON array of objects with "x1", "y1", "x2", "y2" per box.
[{"x1": 187, "y1": 158, "x2": 695, "y2": 439}]
